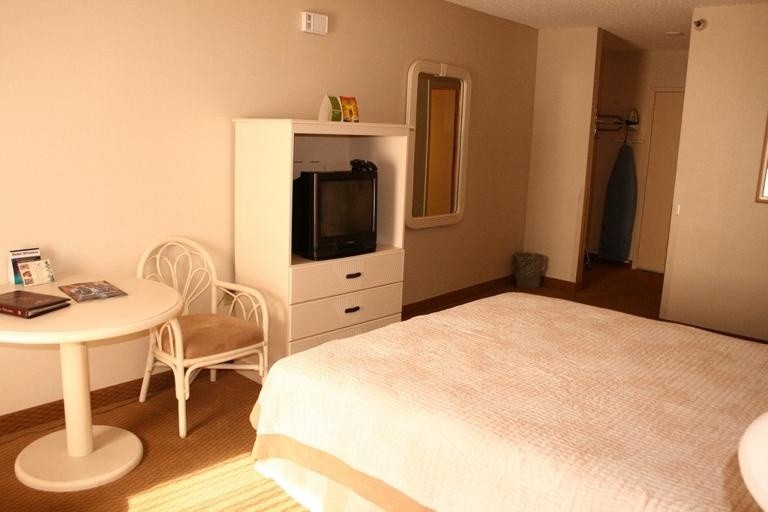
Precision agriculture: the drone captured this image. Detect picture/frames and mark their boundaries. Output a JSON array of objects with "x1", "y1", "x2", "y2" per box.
[{"x1": 753, "y1": 111, "x2": 768, "y2": 204}]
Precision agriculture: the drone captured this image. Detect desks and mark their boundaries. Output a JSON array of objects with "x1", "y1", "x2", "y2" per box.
[{"x1": 0, "y1": 269, "x2": 185, "y2": 493}]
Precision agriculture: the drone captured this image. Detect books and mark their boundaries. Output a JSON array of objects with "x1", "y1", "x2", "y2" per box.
[{"x1": 0, "y1": 290, "x2": 71, "y2": 319}]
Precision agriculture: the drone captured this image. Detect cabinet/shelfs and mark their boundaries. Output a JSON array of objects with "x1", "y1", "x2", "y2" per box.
[{"x1": 227, "y1": 116, "x2": 417, "y2": 391}]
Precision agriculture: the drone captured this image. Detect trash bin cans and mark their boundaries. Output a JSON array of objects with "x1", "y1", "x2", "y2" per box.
[{"x1": 512, "y1": 252, "x2": 544, "y2": 288}]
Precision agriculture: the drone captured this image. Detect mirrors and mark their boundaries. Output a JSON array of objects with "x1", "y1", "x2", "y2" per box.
[{"x1": 403, "y1": 56, "x2": 470, "y2": 230}]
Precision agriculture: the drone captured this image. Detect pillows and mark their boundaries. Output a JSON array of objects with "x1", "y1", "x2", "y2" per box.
[{"x1": 734, "y1": 408, "x2": 768, "y2": 512}]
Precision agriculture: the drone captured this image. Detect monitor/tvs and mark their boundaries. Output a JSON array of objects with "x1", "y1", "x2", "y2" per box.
[{"x1": 293, "y1": 170, "x2": 378, "y2": 261}]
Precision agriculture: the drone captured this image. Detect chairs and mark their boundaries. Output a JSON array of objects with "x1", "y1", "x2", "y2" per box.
[{"x1": 136, "y1": 235, "x2": 270, "y2": 442}]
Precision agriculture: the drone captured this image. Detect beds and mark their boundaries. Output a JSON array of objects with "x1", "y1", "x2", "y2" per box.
[{"x1": 243, "y1": 287, "x2": 768, "y2": 511}]
